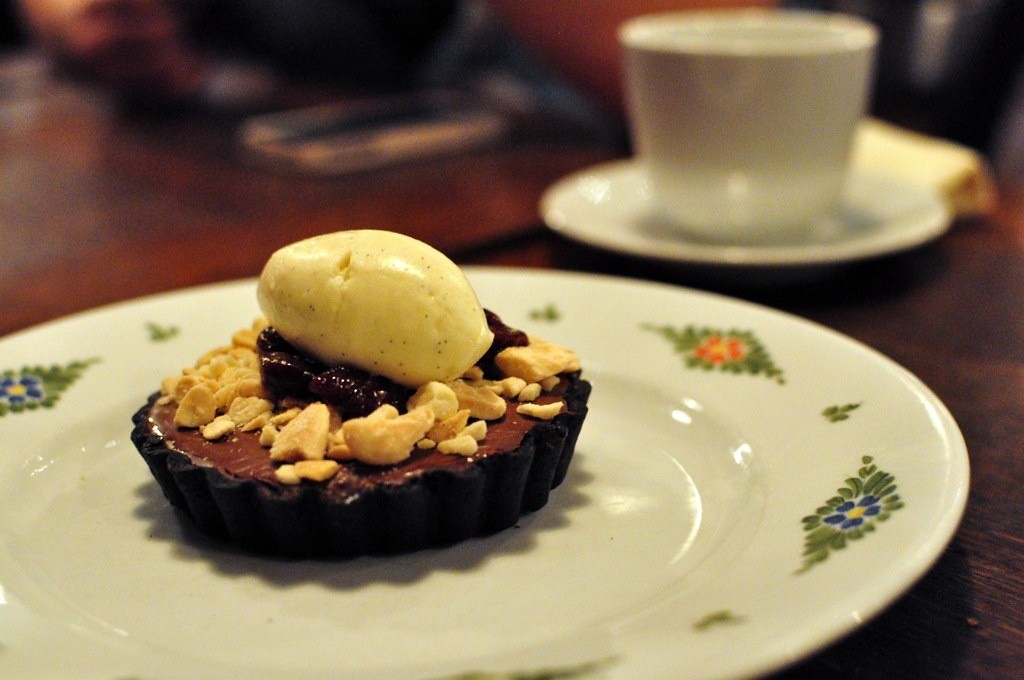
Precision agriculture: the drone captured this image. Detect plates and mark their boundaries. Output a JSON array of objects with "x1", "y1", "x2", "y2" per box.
[
  {"x1": 0, "y1": 261, "x2": 974, "y2": 680},
  {"x1": 538, "y1": 127, "x2": 977, "y2": 268}
]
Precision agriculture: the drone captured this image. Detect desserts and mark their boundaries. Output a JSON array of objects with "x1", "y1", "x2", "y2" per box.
[{"x1": 128, "y1": 230, "x2": 593, "y2": 562}]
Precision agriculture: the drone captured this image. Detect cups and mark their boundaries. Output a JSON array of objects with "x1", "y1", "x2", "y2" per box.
[{"x1": 618, "y1": 7, "x2": 879, "y2": 247}]
